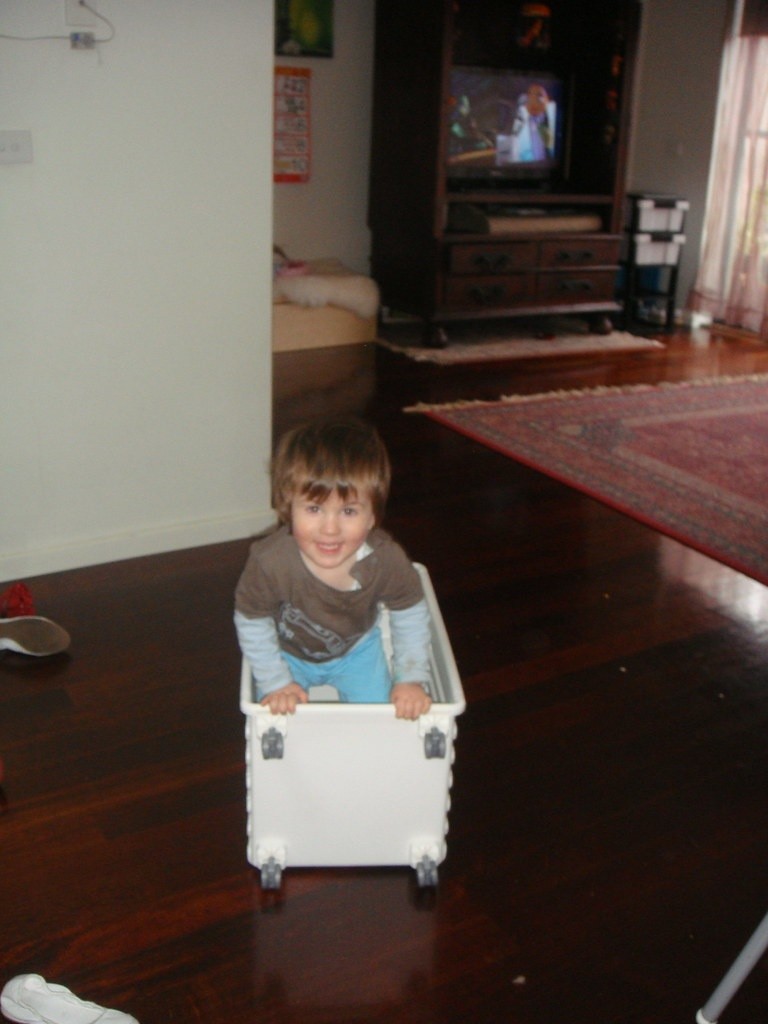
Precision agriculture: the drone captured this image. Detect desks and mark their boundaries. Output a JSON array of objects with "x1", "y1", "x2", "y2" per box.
[{"x1": 273, "y1": 256, "x2": 378, "y2": 353}]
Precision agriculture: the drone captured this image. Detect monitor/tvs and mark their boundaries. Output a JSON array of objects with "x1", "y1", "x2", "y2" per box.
[{"x1": 444, "y1": 66, "x2": 573, "y2": 194}]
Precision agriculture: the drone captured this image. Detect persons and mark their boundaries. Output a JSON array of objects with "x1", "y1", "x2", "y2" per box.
[
  {"x1": 233, "y1": 416, "x2": 434, "y2": 720},
  {"x1": 447, "y1": 82, "x2": 557, "y2": 167}
]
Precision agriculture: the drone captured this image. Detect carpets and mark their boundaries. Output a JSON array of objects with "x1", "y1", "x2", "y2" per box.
[
  {"x1": 376, "y1": 321, "x2": 668, "y2": 365},
  {"x1": 403, "y1": 371, "x2": 768, "y2": 587}
]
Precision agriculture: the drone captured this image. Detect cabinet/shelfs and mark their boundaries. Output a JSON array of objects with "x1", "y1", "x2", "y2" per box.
[
  {"x1": 366, "y1": 0, "x2": 647, "y2": 348},
  {"x1": 622, "y1": 189, "x2": 689, "y2": 336}
]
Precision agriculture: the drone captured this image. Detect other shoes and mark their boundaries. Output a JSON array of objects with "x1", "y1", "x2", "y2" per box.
[
  {"x1": 0, "y1": 973, "x2": 138, "y2": 1024},
  {"x1": 0, "y1": 613, "x2": 73, "y2": 656}
]
